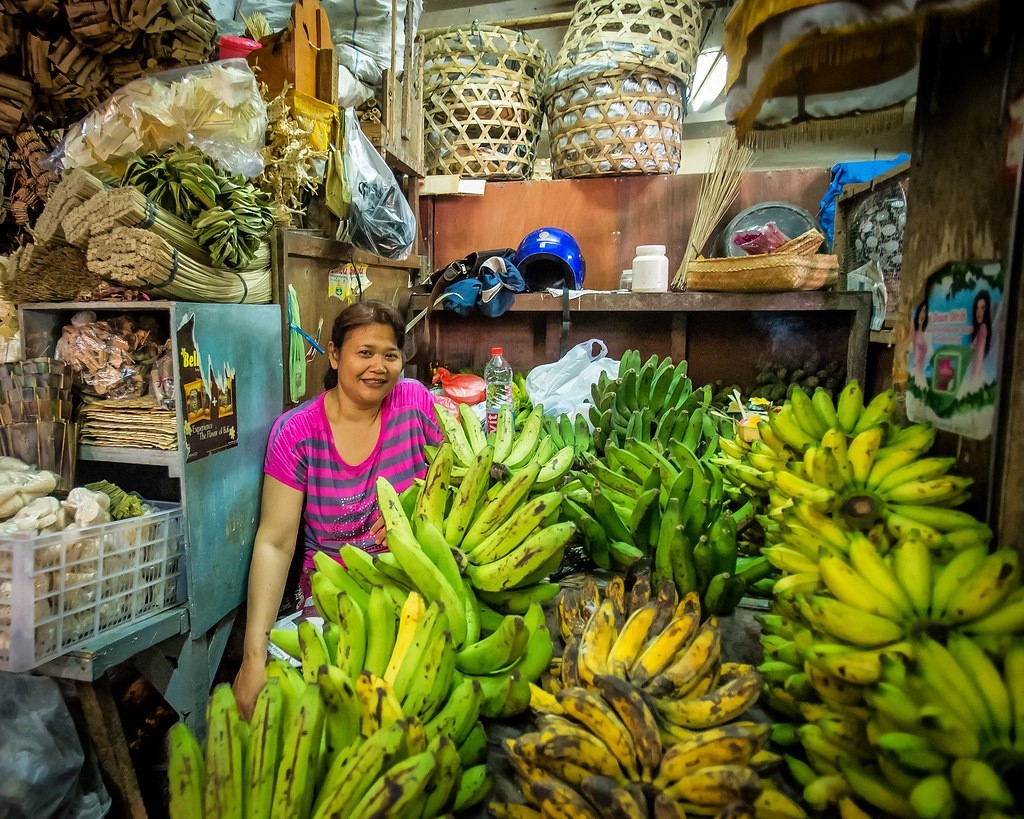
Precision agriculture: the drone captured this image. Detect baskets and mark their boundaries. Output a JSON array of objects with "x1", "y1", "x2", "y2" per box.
[
  {"x1": 687, "y1": 252, "x2": 838, "y2": 291},
  {"x1": 540, "y1": 0, "x2": 702, "y2": 179},
  {"x1": 413, "y1": 20, "x2": 553, "y2": 181}
]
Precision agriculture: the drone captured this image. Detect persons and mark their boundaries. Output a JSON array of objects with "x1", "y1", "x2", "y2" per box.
[{"x1": 231, "y1": 299, "x2": 444, "y2": 719}]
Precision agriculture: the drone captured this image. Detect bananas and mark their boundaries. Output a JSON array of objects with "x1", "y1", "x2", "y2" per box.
[{"x1": 168, "y1": 350, "x2": 1024, "y2": 819}]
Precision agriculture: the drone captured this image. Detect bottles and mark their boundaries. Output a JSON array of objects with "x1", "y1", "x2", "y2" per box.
[
  {"x1": 484, "y1": 347, "x2": 513, "y2": 434},
  {"x1": 632, "y1": 245, "x2": 669, "y2": 293}
]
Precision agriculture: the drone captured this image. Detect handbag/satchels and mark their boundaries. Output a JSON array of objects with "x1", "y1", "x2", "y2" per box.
[
  {"x1": 325, "y1": 106, "x2": 416, "y2": 261},
  {"x1": 431, "y1": 367, "x2": 487, "y2": 406},
  {"x1": 524, "y1": 339, "x2": 621, "y2": 436},
  {"x1": 430, "y1": 248, "x2": 517, "y2": 292}
]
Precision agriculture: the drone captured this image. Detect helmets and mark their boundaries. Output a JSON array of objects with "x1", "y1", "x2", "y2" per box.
[{"x1": 511, "y1": 227, "x2": 586, "y2": 291}]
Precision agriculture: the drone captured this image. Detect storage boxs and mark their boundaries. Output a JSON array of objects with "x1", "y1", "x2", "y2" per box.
[{"x1": 0, "y1": 494, "x2": 180, "y2": 672}]
